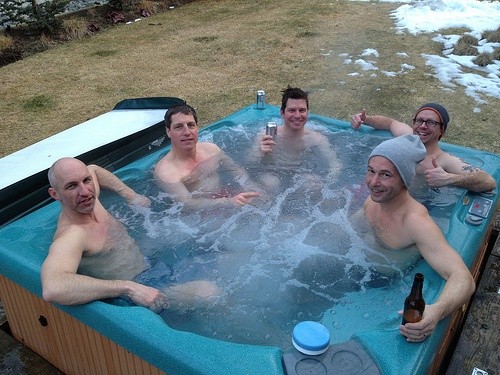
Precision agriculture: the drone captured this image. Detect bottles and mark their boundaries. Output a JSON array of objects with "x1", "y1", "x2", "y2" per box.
[{"x1": 401, "y1": 272, "x2": 425, "y2": 338}]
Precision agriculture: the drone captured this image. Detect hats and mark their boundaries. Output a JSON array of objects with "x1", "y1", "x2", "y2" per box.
[
  {"x1": 415, "y1": 103, "x2": 450, "y2": 140},
  {"x1": 369, "y1": 134, "x2": 427, "y2": 189}
]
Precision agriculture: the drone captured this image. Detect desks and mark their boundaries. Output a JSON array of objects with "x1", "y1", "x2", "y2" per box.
[{"x1": 0, "y1": 97, "x2": 187, "y2": 212}]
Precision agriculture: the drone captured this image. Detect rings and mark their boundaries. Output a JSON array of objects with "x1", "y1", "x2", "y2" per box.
[{"x1": 424, "y1": 333, "x2": 425, "y2": 336}]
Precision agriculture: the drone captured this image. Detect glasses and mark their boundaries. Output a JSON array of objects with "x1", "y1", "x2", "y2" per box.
[{"x1": 412, "y1": 118, "x2": 442, "y2": 128}]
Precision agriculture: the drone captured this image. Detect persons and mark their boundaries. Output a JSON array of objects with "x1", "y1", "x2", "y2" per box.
[
  {"x1": 39, "y1": 156, "x2": 158, "y2": 308},
  {"x1": 275, "y1": 86, "x2": 309, "y2": 135},
  {"x1": 157, "y1": 104, "x2": 220, "y2": 182},
  {"x1": 365, "y1": 133, "x2": 477, "y2": 343},
  {"x1": 349, "y1": 102, "x2": 497, "y2": 193}
]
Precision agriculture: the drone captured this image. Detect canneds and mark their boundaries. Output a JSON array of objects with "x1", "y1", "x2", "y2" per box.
[
  {"x1": 266, "y1": 122, "x2": 277, "y2": 141},
  {"x1": 257, "y1": 90, "x2": 265, "y2": 109}
]
